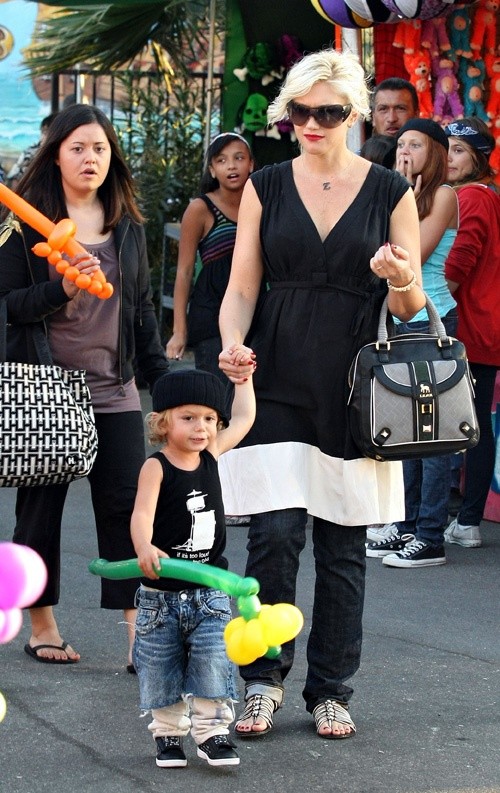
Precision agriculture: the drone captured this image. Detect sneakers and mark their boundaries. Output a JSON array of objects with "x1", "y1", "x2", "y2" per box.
[
  {"x1": 365, "y1": 530, "x2": 414, "y2": 558},
  {"x1": 443, "y1": 516, "x2": 482, "y2": 548},
  {"x1": 381, "y1": 533, "x2": 447, "y2": 568},
  {"x1": 155, "y1": 736, "x2": 187, "y2": 767},
  {"x1": 197, "y1": 735, "x2": 240, "y2": 766},
  {"x1": 366, "y1": 523, "x2": 398, "y2": 541}
]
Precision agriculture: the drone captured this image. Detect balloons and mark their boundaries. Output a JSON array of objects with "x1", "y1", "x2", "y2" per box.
[
  {"x1": 0, "y1": 539, "x2": 49, "y2": 646},
  {"x1": 0, "y1": 692, "x2": 7, "y2": 724},
  {"x1": 0, "y1": 182, "x2": 114, "y2": 301},
  {"x1": 90, "y1": 554, "x2": 305, "y2": 665}
]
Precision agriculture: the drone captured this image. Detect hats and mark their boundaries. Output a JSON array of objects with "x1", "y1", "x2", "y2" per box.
[
  {"x1": 152, "y1": 369, "x2": 226, "y2": 420},
  {"x1": 395, "y1": 118, "x2": 449, "y2": 153}
]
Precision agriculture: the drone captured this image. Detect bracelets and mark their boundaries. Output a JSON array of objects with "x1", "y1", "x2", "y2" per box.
[{"x1": 386, "y1": 271, "x2": 416, "y2": 293}]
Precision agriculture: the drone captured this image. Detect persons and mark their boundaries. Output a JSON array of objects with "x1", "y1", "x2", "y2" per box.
[
  {"x1": 132, "y1": 345, "x2": 257, "y2": 767},
  {"x1": 0, "y1": 104, "x2": 171, "y2": 675},
  {"x1": 7, "y1": 113, "x2": 59, "y2": 193},
  {"x1": 163, "y1": 132, "x2": 262, "y2": 388},
  {"x1": 218, "y1": 48, "x2": 426, "y2": 738},
  {"x1": 359, "y1": 77, "x2": 500, "y2": 568}
]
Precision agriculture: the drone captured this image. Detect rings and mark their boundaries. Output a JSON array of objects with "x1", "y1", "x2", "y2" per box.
[{"x1": 376, "y1": 266, "x2": 382, "y2": 270}]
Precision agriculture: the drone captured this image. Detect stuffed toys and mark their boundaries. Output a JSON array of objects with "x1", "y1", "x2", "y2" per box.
[{"x1": 392, "y1": 0, "x2": 500, "y2": 187}]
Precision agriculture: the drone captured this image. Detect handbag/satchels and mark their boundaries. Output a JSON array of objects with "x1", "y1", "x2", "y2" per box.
[
  {"x1": 0, "y1": 295, "x2": 98, "y2": 486},
  {"x1": 345, "y1": 290, "x2": 480, "y2": 463}
]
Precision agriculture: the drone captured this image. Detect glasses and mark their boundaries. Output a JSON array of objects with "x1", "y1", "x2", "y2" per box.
[{"x1": 288, "y1": 101, "x2": 352, "y2": 129}]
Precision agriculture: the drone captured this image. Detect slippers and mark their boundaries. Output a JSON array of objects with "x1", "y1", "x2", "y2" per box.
[
  {"x1": 127, "y1": 665, "x2": 137, "y2": 673},
  {"x1": 25, "y1": 641, "x2": 77, "y2": 663}
]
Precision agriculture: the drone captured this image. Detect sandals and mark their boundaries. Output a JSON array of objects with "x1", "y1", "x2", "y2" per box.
[
  {"x1": 233, "y1": 695, "x2": 274, "y2": 736},
  {"x1": 313, "y1": 700, "x2": 357, "y2": 738}
]
